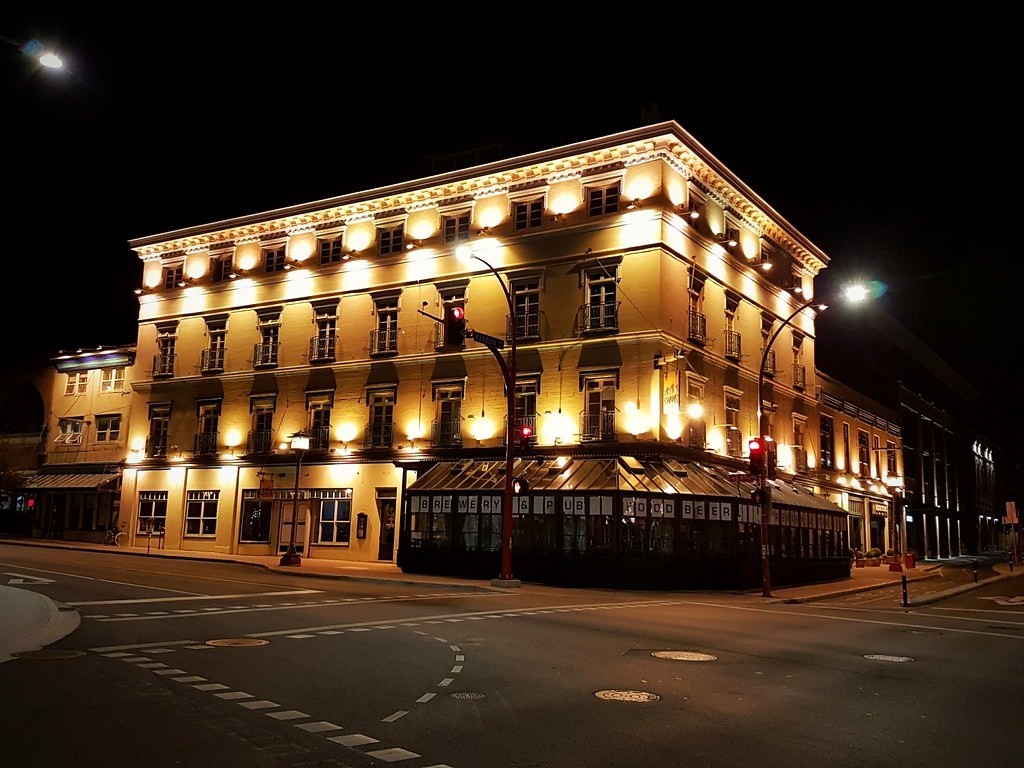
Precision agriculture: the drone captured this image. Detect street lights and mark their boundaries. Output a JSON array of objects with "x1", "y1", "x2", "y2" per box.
[
  {"x1": 279, "y1": 429, "x2": 315, "y2": 566},
  {"x1": 758, "y1": 278, "x2": 870, "y2": 597},
  {"x1": 456, "y1": 244, "x2": 517, "y2": 580}
]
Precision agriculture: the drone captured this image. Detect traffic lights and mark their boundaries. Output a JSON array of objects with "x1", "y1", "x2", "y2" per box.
[
  {"x1": 748, "y1": 439, "x2": 763, "y2": 476},
  {"x1": 751, "y1": 489, "x2": 766, "y2": 508},
  {"x1": 512, "y1": 478, "x2": 529, "y2": 496},
  {"x1": 519, "y1": 424, "x2": 530, "y2": 451},
  {"x1": 443, "y1": 302, "x2": 467, "y2": 351}
]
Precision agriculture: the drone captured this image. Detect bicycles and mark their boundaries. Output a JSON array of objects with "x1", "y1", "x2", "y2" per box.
[{"x1": 101, "y1": 520, "x2": 130, "y2": 547}]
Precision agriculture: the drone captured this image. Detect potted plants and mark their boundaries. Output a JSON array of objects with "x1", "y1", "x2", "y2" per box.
[{"x1": 849, "y1": 547, "x2": 918, "y2": 570}]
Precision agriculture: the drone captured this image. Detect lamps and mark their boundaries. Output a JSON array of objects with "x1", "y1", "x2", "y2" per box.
[
  {"x1": 132, "y1": 202, "x2": 642, "y2": 296},
  {"x1": 677, "y1": 211, "x2": 700, "y2": 218},
  {"x1": 717, "y1": 237, "x2": 827, "y2": 311},
  {"x1": 59, "y1": 345, "x2": 117, "y2": 354}
]
